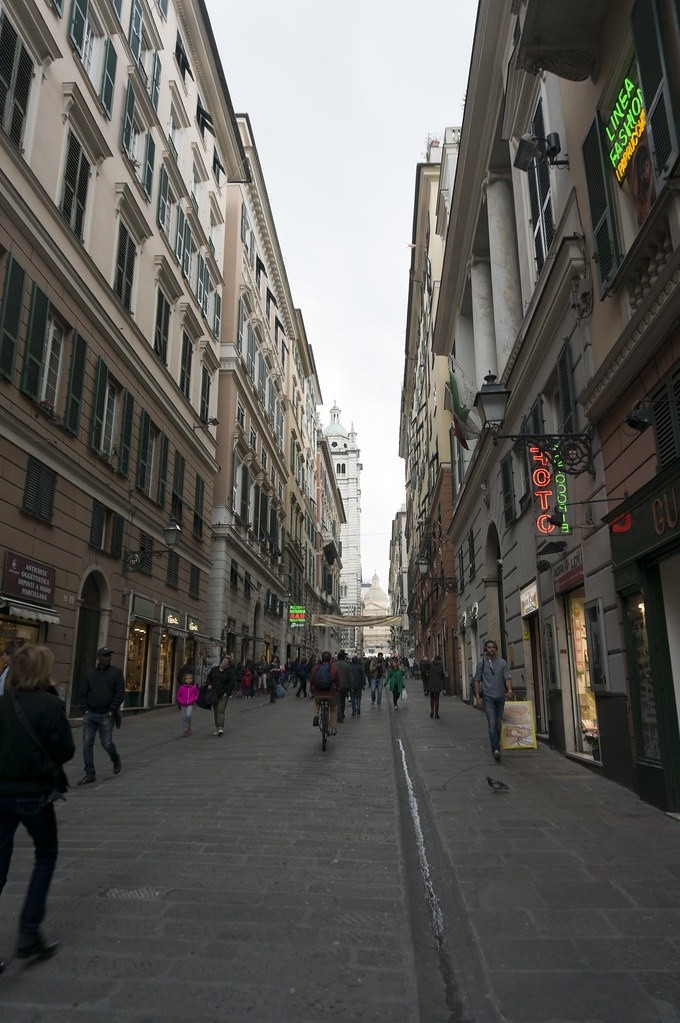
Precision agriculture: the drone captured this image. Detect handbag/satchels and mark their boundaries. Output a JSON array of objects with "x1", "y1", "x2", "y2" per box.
[
  {"x1": 364, "y1": 660, "x2": 371, "y2": 676},
  {"x1": 470, "y1": 658, "x2": 485, "y2": 698},
  {"x1": 501, "y1": 692, "x2": 538, "y2": 751},
  {"x1": 196, "y1": 685, "x2": 212, "y2": 710},
  {"x1": 276, "y1": 680, "x2": 285, "y2": 698},
  {"x1": 114, "y1": 709, "x2": 122, "y2": 729},
  {"x1": 38, "y1": 759, "x2": 71, "y2": 807},
  {"x1": 255, "y1": 663, "x2": 265, "y2": 676},
  {"x1": 401, "y1": 687, "x2": 408, "y2": 703}
]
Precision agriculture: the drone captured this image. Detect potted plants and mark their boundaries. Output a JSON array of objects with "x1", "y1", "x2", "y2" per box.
[{"x1": 584, "y1": 735, "x2": 600, "y2": 760}]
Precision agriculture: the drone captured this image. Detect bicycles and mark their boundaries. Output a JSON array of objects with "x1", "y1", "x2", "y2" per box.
[{"x1": 317, "y1": 699, "x2": 333, "y2": 753}]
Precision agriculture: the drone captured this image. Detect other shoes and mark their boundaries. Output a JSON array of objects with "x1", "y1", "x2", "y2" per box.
[
  {"x1": 212, "y1": 728, "x2": 224, "y2": 736},
  {"x1": 424, "y1": 690, "x2": 429, "y2": 696},
  {"x1": 371, "y1": 702, "x2": 382, "y2": 710},
  {"x1": 112, "y1": 756, "x2": 121, "y2": 774},
  {"x1": 331, "y1": 728, "x2": 336, "y2": 736},
  {"x1": 17, "y1": 932, "x2": 60, "y2": 960},
  {"x1": 392, "y1": 704, "x2": 398, "y2": 710},
  {"x1": 76, "y1": 773, "x2": 96, "y2": 785},
  {"x1": 336, "y1": 715, "x2": 345, "y2": 722},
  {"x1": 269, "y1": 698, "x2": 276, "y2": 703},
  {"x1": 313, "y1": 716, "x2": 320, "y2": 727},
  {"x1": 492, "y1": 751, "x2": 504, "y2": 762},
  {"x1": 233, "y1": 693, "x2": 256, "y2": 700},
  {"x1": 352, "y1": 711, "x2": 360, "y2": 717},
  {"x1": 183, "y1": 728, "x2": 191, "y2": 737},
  {"x1": 429, "y1": 713, "x2": 441, "y2": 718},
  {"x1": 295, "y1": 694, "x2": 308, "y2": 698},
  {"x1": 0, "y1": 962, "x2": 8, "y2": 972}
]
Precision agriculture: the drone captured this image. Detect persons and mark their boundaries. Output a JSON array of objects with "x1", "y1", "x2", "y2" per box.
[
  {"x1": 473, "y1": 640, "x2": 513, "y2": 760},
  {"x1": 421, "y1": 657, "x2": 431, "y2": 696},
  {"x1": 428, "y1": 655, "x2": 446, "y2": 718},
  {"x1": 206, "y1": 655, "x2": 235, "y2": 735},
  {"x1": 0, "y1": 644, "x2": 75, "y2": 973},
  {"x1": 80, "y1": 647, "x2": 126, "y2": 784},
  {"x1": 176, "y1": 649, "x2": 421, "y2": 737},
  {"x1": 0, "y1": 648, "x2": 12, "y2": 695}
]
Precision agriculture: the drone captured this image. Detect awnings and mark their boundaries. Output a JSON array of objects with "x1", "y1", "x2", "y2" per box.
[{"x1": 0, "y1": 596, "x2": 60, "y2": 625}]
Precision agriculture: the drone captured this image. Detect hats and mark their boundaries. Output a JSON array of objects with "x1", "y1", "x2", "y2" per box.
[{"x1": 95, "y1": 647, "x2": 114, "y2": 657}]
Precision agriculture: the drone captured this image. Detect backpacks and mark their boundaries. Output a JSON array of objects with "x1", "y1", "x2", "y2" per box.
[{"x1": 313, "y1": 663, "x2": 332, "y2": 689}]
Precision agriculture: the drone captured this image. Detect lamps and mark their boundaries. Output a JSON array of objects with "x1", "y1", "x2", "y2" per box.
[
  {"x1": 537, "y1": 541, "x2": 567, "y2": 555},
  {"x1": 231, "y1": 522, "x2": 298, "y2": 584},
  {"x1": 264, "y1": 591, "x2": 292, "y2": 617},
  {"x1": 623, "y1": 399, "x2": 680, "y2": 434},
  {"x1": 513, "y1": 132, "x2": 571, "y2": 172},
  {"x1": 122, "y1": 518, "x2": 183, "y2": 576},
  {"x1": 550, "y1": 492, "x2": 628, "y2": 529},
  {"x1": 415, "y1": 554, "x2": 458, "y2": 593},
  {"x1": 473, "y1": 370, "x2": 597, "y2": 481},
  {"x1": 192, "y1": 416, "x2": 219, "y2": 431}
]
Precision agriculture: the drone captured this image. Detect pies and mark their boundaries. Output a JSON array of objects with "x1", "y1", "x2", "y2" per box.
[{"x1": 504, "y1": 704, "x2": 529, "y2": 723}]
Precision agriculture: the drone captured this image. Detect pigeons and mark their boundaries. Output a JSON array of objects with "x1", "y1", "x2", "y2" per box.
[{"x1": 486, "y1": 776, "x2": 510, "y2": 792}]
